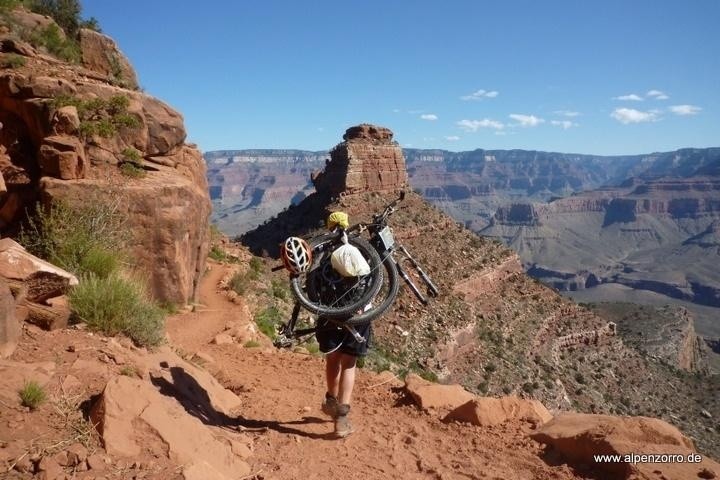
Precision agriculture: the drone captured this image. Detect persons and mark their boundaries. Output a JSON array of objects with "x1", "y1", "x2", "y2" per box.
[{"x1": 304, "y1": 210, "x2": 386, "y2": 440}]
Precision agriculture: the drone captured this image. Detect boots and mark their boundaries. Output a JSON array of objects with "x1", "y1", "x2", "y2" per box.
[
  {"x1": 333, "y1": 402, "x2": 357, "y2": 440},
  {"x1": 321, "y1": 391, "x2": 338, "y2": 420}
]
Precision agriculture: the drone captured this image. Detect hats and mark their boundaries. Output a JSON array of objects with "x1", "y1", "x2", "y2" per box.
[{"x1": 326, "y1": 211, "x2": 349, "y2": 231}]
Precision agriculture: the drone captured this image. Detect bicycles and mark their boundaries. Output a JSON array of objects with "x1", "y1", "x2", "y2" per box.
[{"x1": 272, "y1": 189, "x2": 438, "y2": 355}]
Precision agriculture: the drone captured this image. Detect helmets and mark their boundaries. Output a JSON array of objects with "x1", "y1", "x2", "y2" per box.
[{"x1": 278, "y1": 236, "x2": 313, "y2": 276}]
